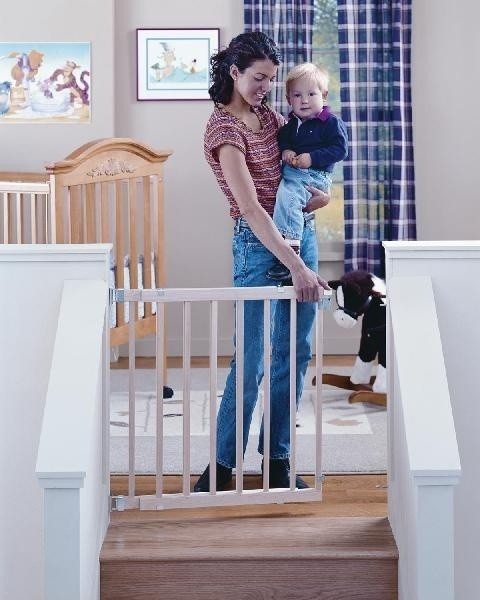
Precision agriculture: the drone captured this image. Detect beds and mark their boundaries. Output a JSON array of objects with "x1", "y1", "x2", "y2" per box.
[{"x1": 0, "y1": 137, "x2": 177, "y2": 406}]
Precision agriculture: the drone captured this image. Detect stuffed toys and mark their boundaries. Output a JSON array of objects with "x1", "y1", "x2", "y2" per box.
[{"x1": 312, "y1": 270, "x2": 387, "y2": 407}]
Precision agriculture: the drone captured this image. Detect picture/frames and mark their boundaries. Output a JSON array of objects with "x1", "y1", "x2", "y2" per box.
[{"x1": 134, "y1": 26, "x2": 222, "y2": 102}]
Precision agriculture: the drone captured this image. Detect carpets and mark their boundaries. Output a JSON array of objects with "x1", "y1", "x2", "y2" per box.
[{"x1": 110, "y1": 364, "x2": 390, "y2": 477}]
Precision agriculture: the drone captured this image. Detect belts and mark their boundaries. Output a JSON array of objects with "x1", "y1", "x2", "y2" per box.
[{"x1": 235, "y1": 220, "x2": 249, "y2": 227}]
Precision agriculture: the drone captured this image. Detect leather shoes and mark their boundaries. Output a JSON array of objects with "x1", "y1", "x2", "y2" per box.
[
  {"x1": 266, "y1": 261, "x2": 292, "y2": 280},
  {"x1": 194, "y1": 463, "x2": 231, "y2": 493},
  {"x1": 262, "y1": 458, "x2": 308, "y2": 489}
]
